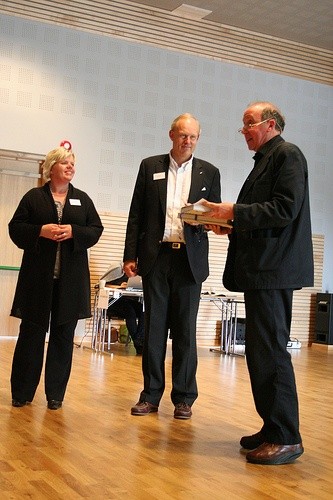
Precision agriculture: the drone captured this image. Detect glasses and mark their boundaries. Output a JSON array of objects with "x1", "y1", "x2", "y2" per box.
[{"x1": 238, "y1": 116, "x2": 275, "y2": 134}]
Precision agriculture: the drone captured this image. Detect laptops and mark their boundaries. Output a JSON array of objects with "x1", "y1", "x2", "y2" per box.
[{"x1": 117, "y1": 275, "x2": 143, "y2": 289}]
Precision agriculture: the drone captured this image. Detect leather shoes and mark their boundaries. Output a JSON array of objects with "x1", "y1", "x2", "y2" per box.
[
  {"x1": 239, "y1": 428, "x2": 267, "y2": 450},
  {"x1": 130, "y1": 401, "x2": 158, "y2": 416},
  {"x1": 246, "y1": 441, "x2": 304, "y2": 466},
  {"x1": 174, "y1": 403, "x2": 193, "y2": 419}
]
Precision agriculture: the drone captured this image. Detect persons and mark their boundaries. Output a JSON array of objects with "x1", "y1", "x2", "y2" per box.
[
  {"x1": 8, "y1": 145, "x2": 104, "y2": 410},
  {"x1": 198, "y1": 102, "x2": 313, "y2": 465},
  {"x1": 94, "y1": 261, "x2": 145, "y2": 354},
  {"x1": 123, "y1": 112, "x2": 222, "y2": 419}
]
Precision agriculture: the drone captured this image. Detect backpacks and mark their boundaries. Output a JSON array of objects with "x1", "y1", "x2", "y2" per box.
[{"x1": 118, "y1": 324, "x2": 132, "y2": 343}]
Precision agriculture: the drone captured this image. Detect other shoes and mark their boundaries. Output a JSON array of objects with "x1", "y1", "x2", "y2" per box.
[
  {"x1": 12, "y1": 398, "x2": 26, "y2": 407},
  {"x1": 134, "y1": 338, "x2": 146, "y2": 356},
  {"x1": 47, "y1": 399, "x2": 63, "y2": 409}
]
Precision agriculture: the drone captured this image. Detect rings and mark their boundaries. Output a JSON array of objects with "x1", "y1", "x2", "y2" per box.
[{"x1": 53, "y1": 235, "x2": 57, "y2": 238}]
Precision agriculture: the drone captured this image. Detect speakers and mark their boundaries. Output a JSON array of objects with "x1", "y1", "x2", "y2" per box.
[{"x1": 314, "y1": 293, "x2": 333, "y2": 344}]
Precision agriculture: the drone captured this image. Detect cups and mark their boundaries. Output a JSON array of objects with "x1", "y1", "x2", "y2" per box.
[
  {"x1": 98, "y1": 280, "x2": 105, "y2": 288},
  {"x1": 210, "y1": 288, "x2": 215, "y2": 293}
]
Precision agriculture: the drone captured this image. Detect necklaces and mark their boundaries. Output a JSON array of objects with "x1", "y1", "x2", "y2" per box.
[{"x1": 54, "y1": 193, "x2": 66, "y2": 197}]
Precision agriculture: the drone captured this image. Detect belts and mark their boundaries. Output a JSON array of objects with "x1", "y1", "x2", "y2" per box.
[{"x1": 159, "y1": 241, "x2": 187, "y2": 252}]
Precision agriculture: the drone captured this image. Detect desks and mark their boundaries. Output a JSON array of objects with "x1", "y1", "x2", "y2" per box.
[{"x1": 82, "y1": 289, "x2": 246, "y2": 357}]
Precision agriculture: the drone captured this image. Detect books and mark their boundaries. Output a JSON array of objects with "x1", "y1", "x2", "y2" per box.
[{"x1": 180, "y1": 198, "x2": 233, "y2": 228}]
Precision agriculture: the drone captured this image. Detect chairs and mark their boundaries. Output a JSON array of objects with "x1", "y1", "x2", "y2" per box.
[{"x1": 92, "y1": 302, "x2": 129, "y2": 350}]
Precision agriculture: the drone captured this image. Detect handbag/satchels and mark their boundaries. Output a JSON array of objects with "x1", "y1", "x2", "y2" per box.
[{"x1": 105, "y1": 327, "x2": 118, "y2": 342}]
